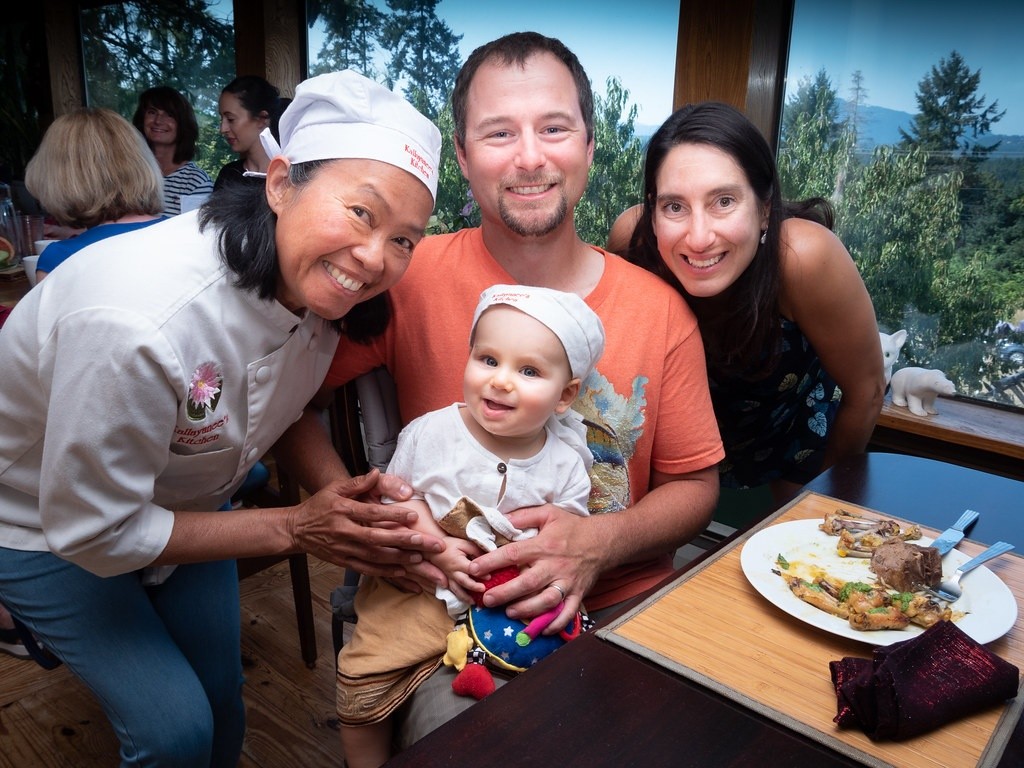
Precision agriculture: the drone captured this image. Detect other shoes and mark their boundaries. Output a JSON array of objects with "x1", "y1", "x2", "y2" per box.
[{"x1": 0, "y1": 626, "x2": 43, "y2": 660}]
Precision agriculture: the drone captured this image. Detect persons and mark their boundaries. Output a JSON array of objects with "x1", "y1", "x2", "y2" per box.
[
  {"x1": 23, "y1": 74, "x2": 280, "y2": 285},
  {"x1": 604, "y1": 102, "x2": 885, "y2": 503},
  {"x1": 293, "y1": 31, "x2": 726, "y2": 768},
  {"x1": 1, "y1": 70, "x2": 446, "y2": 768}
]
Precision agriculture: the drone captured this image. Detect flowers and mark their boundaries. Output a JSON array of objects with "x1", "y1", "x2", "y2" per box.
[{"x1": 187, "y1": 360, "x2": 223, "y2": 421}]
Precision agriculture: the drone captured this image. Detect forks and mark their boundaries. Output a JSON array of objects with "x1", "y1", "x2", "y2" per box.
[{"x1": 912, "y1": 541, "x2": 1015, "y2": 602}]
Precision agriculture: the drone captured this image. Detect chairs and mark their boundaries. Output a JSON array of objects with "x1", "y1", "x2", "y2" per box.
[
  {"x1": 235, "y1": 457, "x2": 318, "y2": 671},
  {"x1": 330, "y1": 302, "x2": 402, "y2": 659}
]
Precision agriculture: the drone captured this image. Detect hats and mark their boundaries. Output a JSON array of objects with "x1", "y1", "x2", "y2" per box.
[{"x1": 469, "y1": 284, "x2": 604, "y2": 382}]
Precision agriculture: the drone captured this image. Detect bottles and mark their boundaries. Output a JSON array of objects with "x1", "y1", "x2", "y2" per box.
[{"x1": 0, "y1": 182, "x2": 19, "y2": 253}]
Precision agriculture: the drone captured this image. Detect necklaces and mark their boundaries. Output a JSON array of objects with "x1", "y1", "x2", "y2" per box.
[{"x1": 711, "y1": 286, "x2": 758, "y2": 365}]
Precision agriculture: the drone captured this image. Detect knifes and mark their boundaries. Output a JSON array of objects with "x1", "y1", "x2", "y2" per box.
[{"x1": 929, "y1": 510, "x2": 980, "y2": 555}]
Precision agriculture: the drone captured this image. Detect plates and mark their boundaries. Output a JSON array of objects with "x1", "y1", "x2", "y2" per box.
[{"x1": 740, "y1": 518, "x2": 1017, "y2": 647}]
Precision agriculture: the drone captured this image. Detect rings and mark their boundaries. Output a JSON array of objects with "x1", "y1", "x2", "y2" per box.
[{"x1": 550, "y1": 585, "x2": 565, "y2": 599}]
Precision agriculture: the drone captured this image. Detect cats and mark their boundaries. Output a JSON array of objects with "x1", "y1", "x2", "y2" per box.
[{"x1": 879, "y1": 330, "x2": 907, "y2": 385}]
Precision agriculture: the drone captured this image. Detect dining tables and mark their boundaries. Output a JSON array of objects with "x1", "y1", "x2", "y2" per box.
[{"x1": 378, "y1": 452, "x2": 1024, "y2": 768}]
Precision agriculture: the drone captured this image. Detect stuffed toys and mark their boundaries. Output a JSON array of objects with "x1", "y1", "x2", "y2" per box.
[{"x1": 443, "y1": 564, "x2": 595, "y2": 699}]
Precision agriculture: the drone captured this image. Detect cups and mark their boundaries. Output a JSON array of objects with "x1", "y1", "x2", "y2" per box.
[
  {"x1": 22, "y1": 256, "x2": 38, "y2": 289},
  {"x1": 6, "y1": 215, "x2": 44, "y2": 266},
  {"x1": 33, "y1": 240, "x2": 58, "y2": 254}
]
